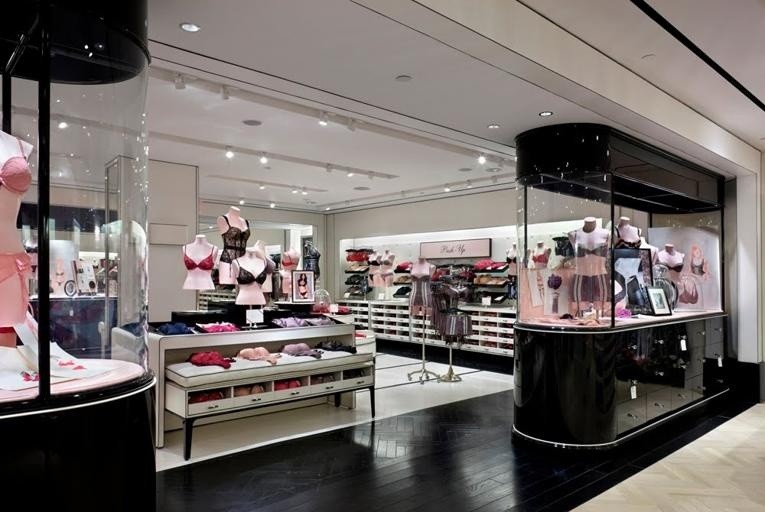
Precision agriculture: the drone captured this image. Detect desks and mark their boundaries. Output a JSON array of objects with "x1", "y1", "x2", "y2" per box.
[
  {"x1": 113, "y1": 323, "x2": 355, "y2": 450},
  {"x1": 164, "y1": 361, "x2": 376, "y2": 461}
]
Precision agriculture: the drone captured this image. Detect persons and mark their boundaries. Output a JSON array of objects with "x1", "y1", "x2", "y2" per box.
[
  {"x1": 532, "y1": 215, "x2": 710, "y2": 316},
  {"x1": 409, "y1": 256, "x2": 436, "y2": 308},
  {"x1": 377, "y1": 249, "x2": 396, "y2": 288},
  {"x1": 0, "y1": 129, "x2": 35, "y2": 326},
  {"x1": 367, "y1": 250, "x2": 382, "y2": 287},
  {"x1": 181, "y1": 206, "x2": 321, "y2": 306},
  {"x1": 504, "y1": 242, "x2": 519, "y2": 277},
  {"x1": 20, "y1": 232, "x2": 39, "y2": 280}
]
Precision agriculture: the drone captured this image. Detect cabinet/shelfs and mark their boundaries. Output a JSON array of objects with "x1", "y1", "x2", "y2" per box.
[
  {"x1": 614, "y1": 317, "x2": 726, "y2": 436},
  {"x1": 345, "y1": 249, "x2": 510, "y2": 298},
  {"x1": 26, "y1": 296, "x2": 117, "y2": 359},
  {"x1": 336, "y1": 300, "x2": 516, "y2": 376}
]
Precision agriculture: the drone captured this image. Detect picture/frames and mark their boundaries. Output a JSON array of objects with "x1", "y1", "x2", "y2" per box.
[
  {"x1": 613, "y1": 248, "x2": 653, "y2": 316},
  {"x1": 645, "y1": 286, "x2": 672, "y2": 315},
  {"x1": 291, "y1": 270, "x2": 315, "y2": 302}
]
